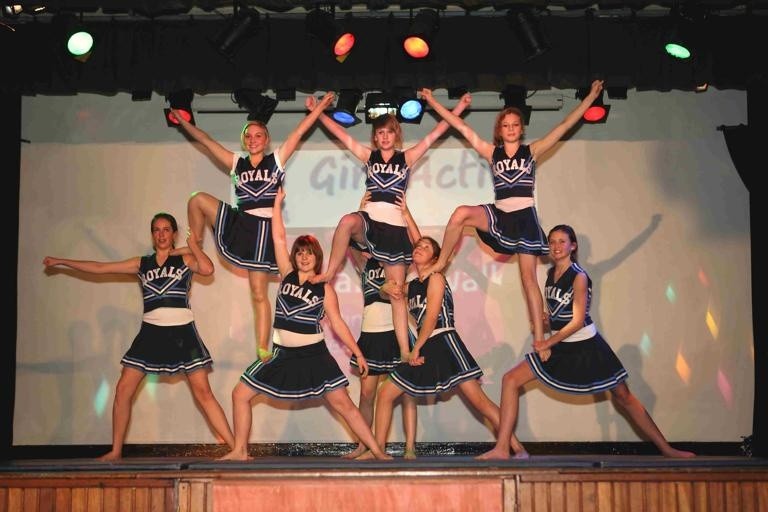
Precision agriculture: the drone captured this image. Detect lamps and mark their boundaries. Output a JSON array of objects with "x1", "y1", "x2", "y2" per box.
[
  {"x1": 403, "y1": 10, "x2": 439, "y2": 57},
  {"x1": 215, "y1": 8, "x2": 260, "y2": 56},
  {"x1": 505, "y1": 4, "x2": 551, "y2": 62},
  {"x1": 664, "y1": 17, "x2": 694, "y2": 60},
  {"x1": 304, "y1": 8, "x2": 355, "y2": 56},
  {"x1": 49, "y1": 14, "x2": 93, "y2": 56}
]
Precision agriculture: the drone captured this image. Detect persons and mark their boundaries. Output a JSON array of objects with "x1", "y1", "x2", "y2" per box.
[
  {"x1": 473, "y1": 224, "x2": 695, "y2": 461},
  {"x1": 419, "y1": 80, "x2": 603, "y2": 362},
  {"x1": 306, "y1": 90, "x2": 473, "y2": 367},
  {"x1": 214, "y1": 186, "x2": 393, "y2": 459},
  {"x1": 43, "y1": 213, "x2": 256, "y2": 462},
  {"x1": 168, "y1": 91, "x2": 335, "y2": 362},
  {"x1": 343, "y1": 257, "x2": 416, "y2": 459},
  {"x1": 356, "y1": 235, "x2": 530, "y2": 459}
]
